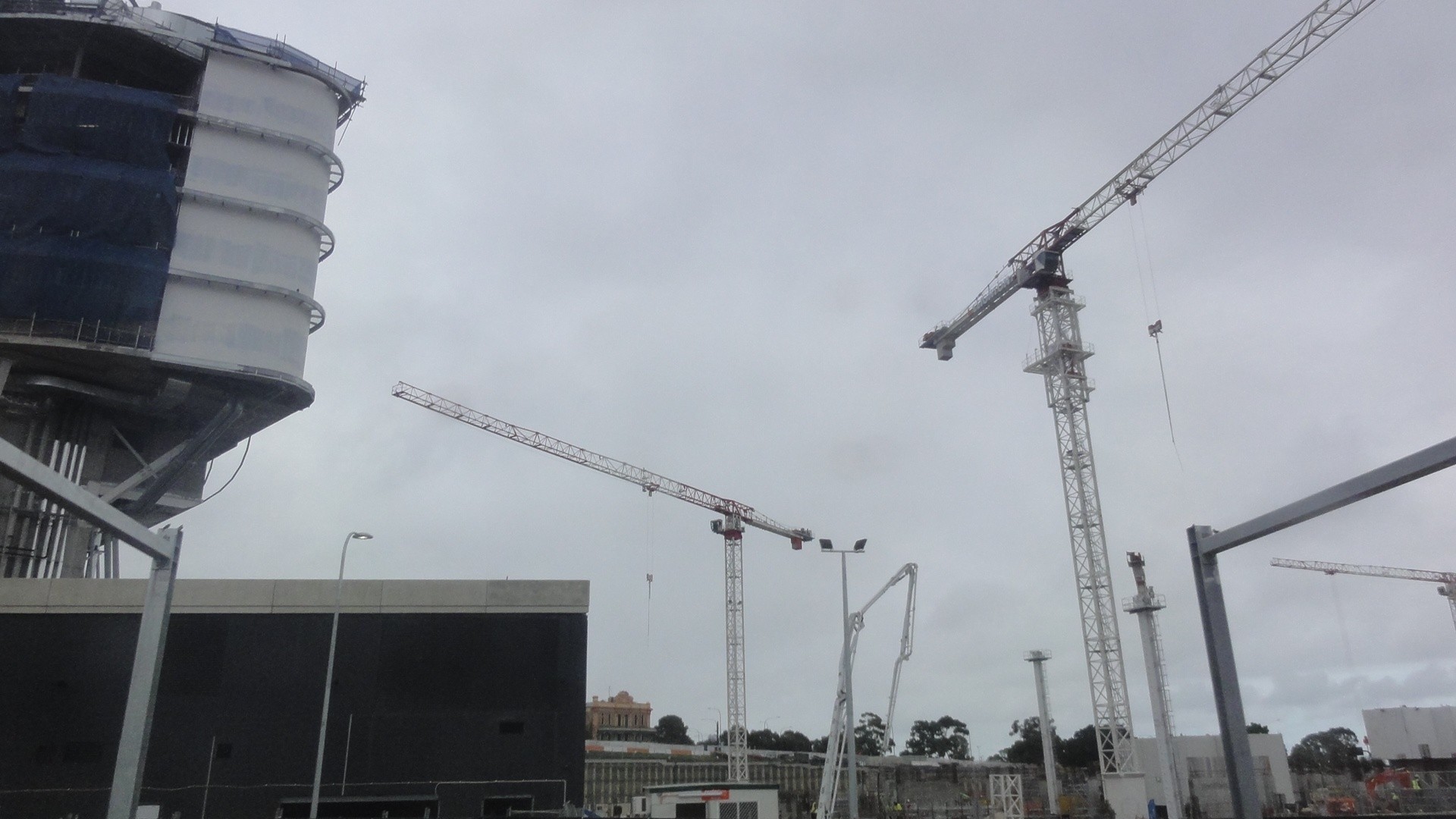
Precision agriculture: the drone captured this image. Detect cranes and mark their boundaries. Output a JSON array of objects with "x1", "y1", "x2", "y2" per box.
[
  {"x1": 919, "y1": 1, "x2": 1380, "y2": 777},
  {"x1": 1268, "y1": 557, "x2": 1456, "y2": 631},
  {"x1": 390, "y1": 380, "x2": 816, "y2": 784}
]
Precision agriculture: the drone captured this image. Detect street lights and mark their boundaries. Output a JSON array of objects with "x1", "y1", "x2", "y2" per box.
[
  {"x1": 310, "y1": 532, "x2": 376, "y2": 819},
  {"x1": 818, "y1": 537, "x2": 867, "y2": 819}
]
